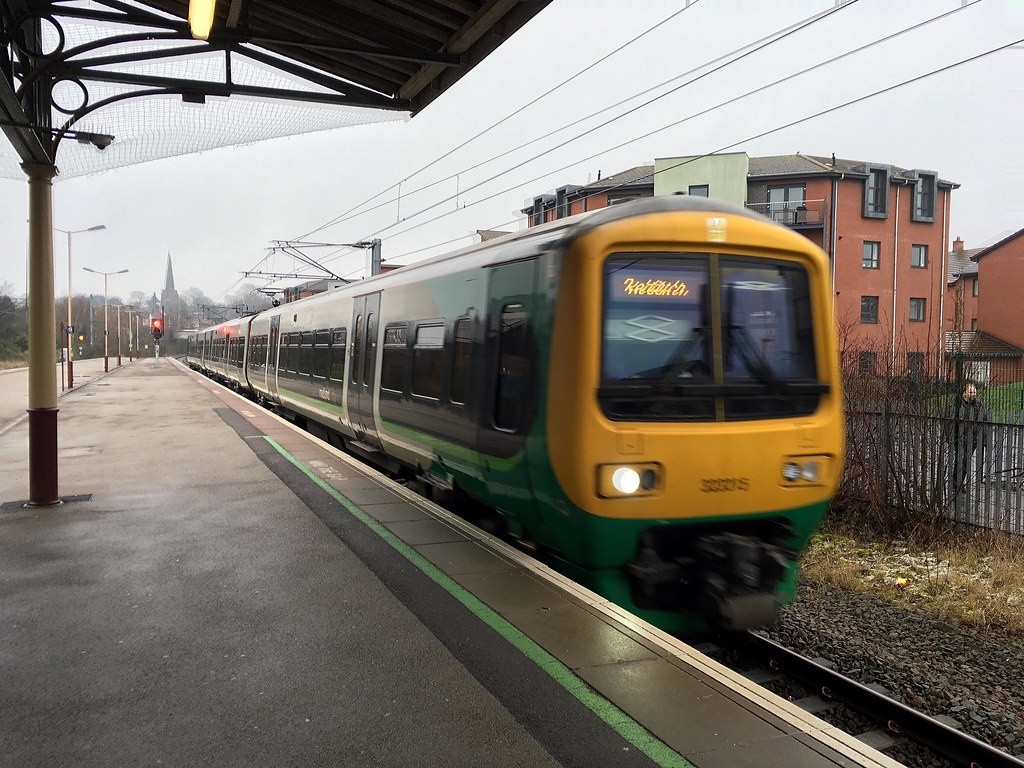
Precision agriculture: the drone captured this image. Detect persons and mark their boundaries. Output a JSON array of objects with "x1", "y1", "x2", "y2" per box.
[{"x1": 944, "y1": 382, "x2": 993, "y2": 493}]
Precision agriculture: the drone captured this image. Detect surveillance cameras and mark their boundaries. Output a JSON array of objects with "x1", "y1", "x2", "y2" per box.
[{"x1": 91, "y1": 135, "x2": 112, "y2": 150}]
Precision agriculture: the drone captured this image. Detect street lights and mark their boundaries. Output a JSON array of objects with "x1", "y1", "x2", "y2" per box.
[
  {"x1": 82, "y1": 267, "x2": 129, "y2": 372},
  {"x1": 27, "y1": 218, "x2": 106, "y2": 388},
  {"x1": 102, "y1": 304, "x2": 143, "y2": 366}
]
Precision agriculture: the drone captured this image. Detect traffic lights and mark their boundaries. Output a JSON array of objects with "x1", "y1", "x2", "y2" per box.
[{"x1": 151, "y1": 318, "x2": 162, "y2": 334}]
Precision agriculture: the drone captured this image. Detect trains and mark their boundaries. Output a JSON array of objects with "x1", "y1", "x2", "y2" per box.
[{"x1": 187, "y1": 197, "x2": 846, "y2": 632}]
